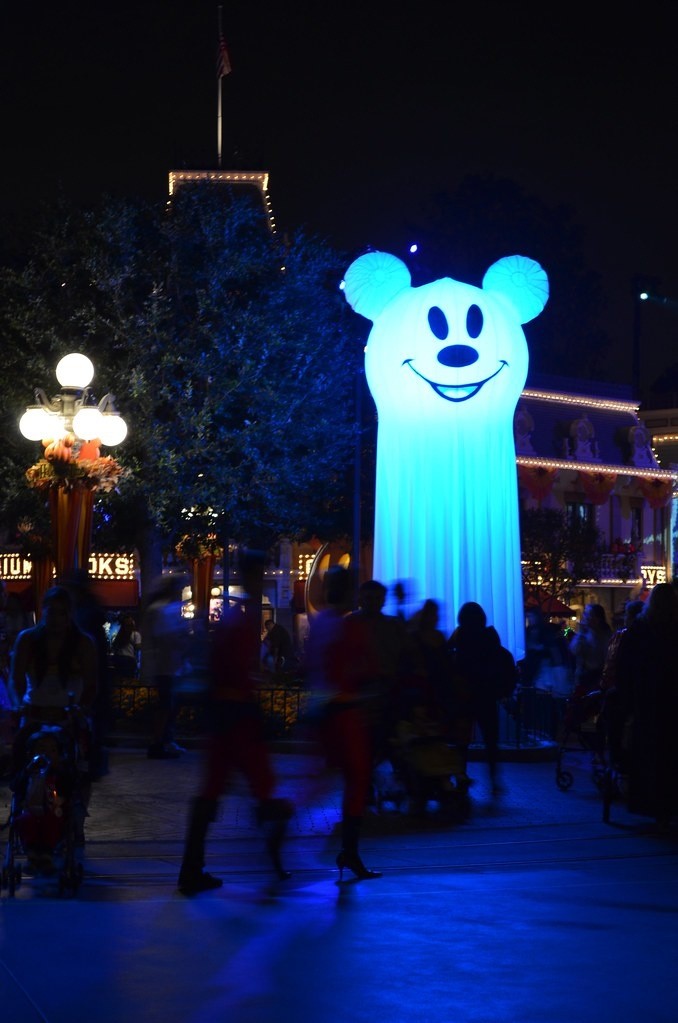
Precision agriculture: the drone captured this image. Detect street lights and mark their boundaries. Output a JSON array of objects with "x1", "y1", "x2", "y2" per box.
[{"x1": 17, "y1": 350, "x2": 128, "y2": 589}]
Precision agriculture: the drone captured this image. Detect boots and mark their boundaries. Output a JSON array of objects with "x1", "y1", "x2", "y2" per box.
[
  {"x1": 259, "y1": 799, "x2": 292, "y2": 877},
  {"x1": 335, "y1": 813, "x2": 385, "y2": 878},
  {"x1": 177, "y1": 799, "x2": 222, "y2": 892}
]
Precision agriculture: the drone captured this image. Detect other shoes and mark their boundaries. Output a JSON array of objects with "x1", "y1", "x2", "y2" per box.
[
  {"x1": 147, "y1": 748, "x2": 179, "y2": 760},
  {"x1": 22, "y1": 855, "x2": 39, "y2": 874},
  {"x1": 40, "y1": 854, "x2": 55, "y2": 874}
]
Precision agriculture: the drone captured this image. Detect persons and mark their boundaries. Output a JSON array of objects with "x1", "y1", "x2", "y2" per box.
[
  {"x1": 322, "y1": 580, "x2": 389, "y2": 878},
  {"x1": 0, "y1": 572, "x2": 678, "y2": 894},
  {"x1": 6, "y1": 733, "x2": 88, "y2": 856},
  {"x1": 10, "y1": 586, "x2": 100, "y2": 878},
  {"x1": 177, "y1": 547, "x2": 307, "y2": 892}
]
